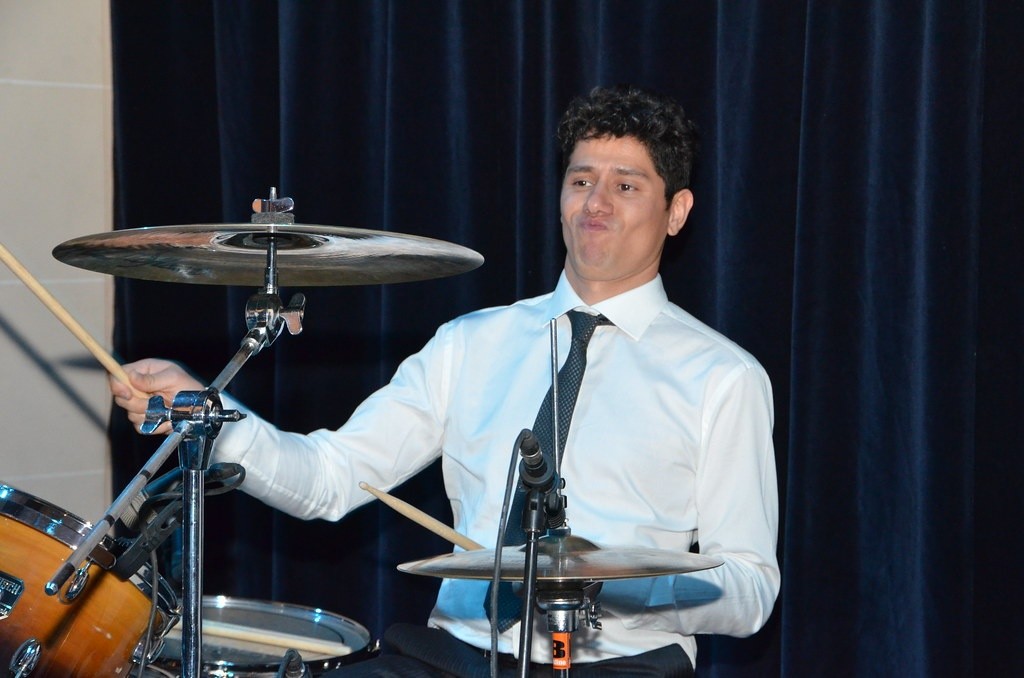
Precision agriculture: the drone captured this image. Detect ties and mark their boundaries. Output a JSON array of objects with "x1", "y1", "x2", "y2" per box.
[{"x1": 483, "y1": 308, "x2": 614, "y2": 633}]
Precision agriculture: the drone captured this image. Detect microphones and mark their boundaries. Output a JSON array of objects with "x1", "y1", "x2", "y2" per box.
[
  {"x1": 120, "y1": 466, "x2": 185, "y2": 531},
  {"x1": 284, "y1": 648, "x2": 312, "y2": 678},
  {"x1": 520, "y1": 430, "x2": 565, "y2": 528}
]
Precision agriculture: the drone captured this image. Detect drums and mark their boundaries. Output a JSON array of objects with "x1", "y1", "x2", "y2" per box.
[
  {"x1": 0, "y1": 481, "x2": 182, "y2": 678},
  {"x1": 126, "y1": 593, "x2": 380, "y2": 678}
]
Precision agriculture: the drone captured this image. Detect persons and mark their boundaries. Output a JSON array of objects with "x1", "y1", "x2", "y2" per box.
[{"x1": 109, "y1": 87, "x2": 781, "y2": 678}]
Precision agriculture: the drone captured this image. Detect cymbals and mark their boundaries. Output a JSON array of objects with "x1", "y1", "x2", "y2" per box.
[
  {"x1": 395, "y1": 537, "x2": 727, "y2": 580},
  {"x1": 50, "y1": 220, "x2": 487, "y2": 289}
]
